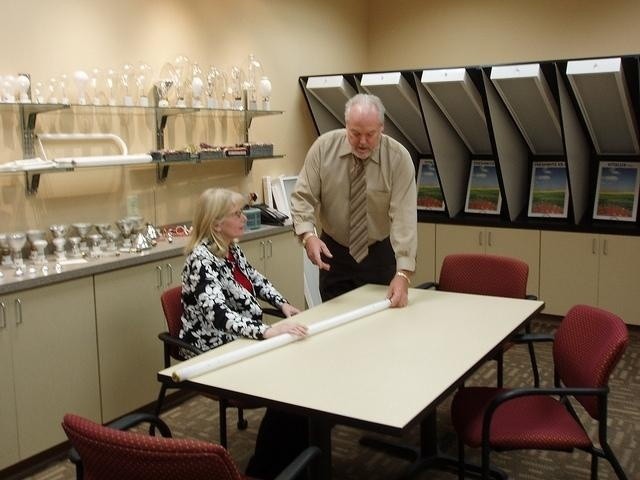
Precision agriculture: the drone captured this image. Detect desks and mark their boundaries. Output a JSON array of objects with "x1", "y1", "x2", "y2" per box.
[{"x1": 149, "y1": 283, "x2": 546, "y2": 478}]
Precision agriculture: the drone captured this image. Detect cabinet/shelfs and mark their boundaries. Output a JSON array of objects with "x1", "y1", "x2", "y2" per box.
[
  {"x1": 407, "y1": 223, "x2": 434, "y2": 289},
  {"x1": 0, "y1": 278, "x2": 112, "y2": 475},
  {"x1": 435, "y1": 220, "x2": 540, "y2": 308},
  {"x1": 93, "y1": 240, "x2": 215, "y2": 423},
  {"x1": 3, "y1": 100, "x2": 285, "y2": 173},
  {"x1": 238, "y1": 226, "x2": 308, "y2": 340},
  {"x1": 540, "y1": 230, "x2": 640, "y2": 328}
]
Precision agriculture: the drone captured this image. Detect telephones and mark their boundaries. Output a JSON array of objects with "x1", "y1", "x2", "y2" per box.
[{"x1": 251, "y1": 203, "x2": 289, "y2": 226}]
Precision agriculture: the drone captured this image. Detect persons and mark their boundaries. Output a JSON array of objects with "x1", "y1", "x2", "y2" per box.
[
  {"x1": 290, "y1": 94, "x2": 419, "y2": 308},
  {"x1": 174, "y1": 185, "x2": 337, "y2": 479}
]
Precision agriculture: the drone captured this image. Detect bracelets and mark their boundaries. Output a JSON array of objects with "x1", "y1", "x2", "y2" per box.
[
  {"x1": 395, "y1": 271, "x2": 410, "y2": 284},
  {"x1": 302, "y1": 232, "x2": 315, "y2": 249}
]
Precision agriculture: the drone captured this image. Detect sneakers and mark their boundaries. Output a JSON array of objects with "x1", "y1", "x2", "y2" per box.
[{"x1": 243, "y1": 454, "x2": 310, "y2": 480}]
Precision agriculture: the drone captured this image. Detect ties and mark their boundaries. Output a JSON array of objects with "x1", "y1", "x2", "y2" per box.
[{"x1": 347, "y1": 153, "x2": 373, "y2": 265}]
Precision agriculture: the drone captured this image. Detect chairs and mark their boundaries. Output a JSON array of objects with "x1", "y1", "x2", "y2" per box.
[
  {"x1": 448, "y1": 301, "x2": 631, "y2": 478},
  {"x1": 147, "y1": 283, "x2": 300, "y2": 436},
  {"x1": 418, "y1": 252, "x2": 546, "y2": 395},
  {"x1": 60, "y1": 404, "x2": 329, "y2": 480}
]
